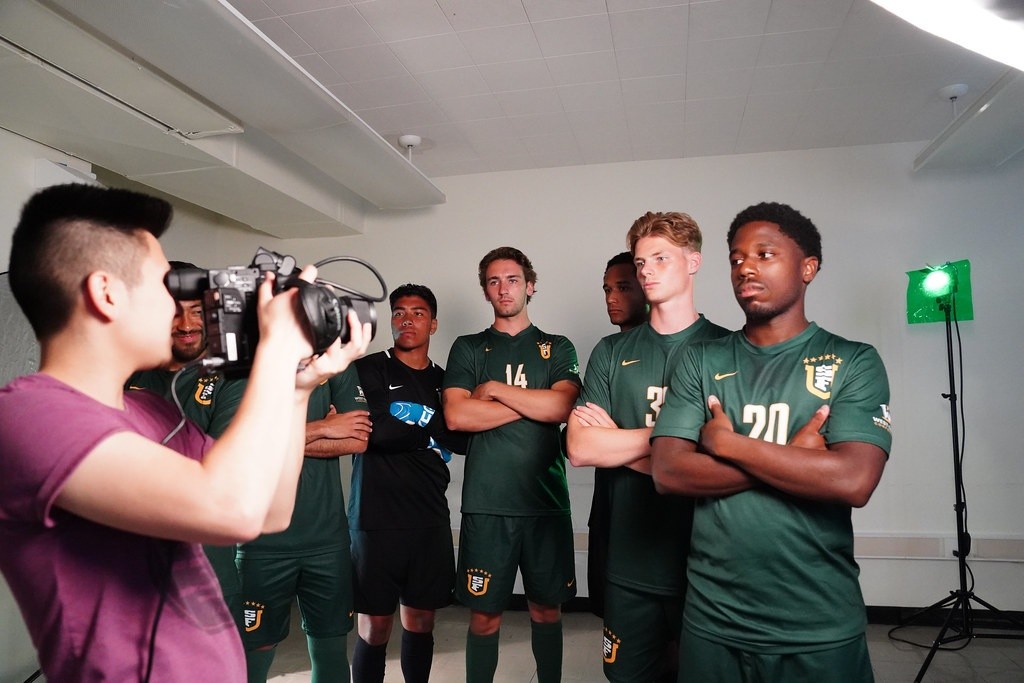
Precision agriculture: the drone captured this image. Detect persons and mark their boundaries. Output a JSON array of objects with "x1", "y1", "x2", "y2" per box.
[
  {"x1": 133, "y1": 261, "x2": 373, "y2": 683},
  {"x1": 569, "y1": 212, "x2": 734, "y2": 683},
  {"x1": 586, "y1": 251, "x2": 651, "y2": 620},
  {"x1": 443, "y1": 246, "x2": 583, "y2": 683},
  {"x1": 651, "y1": 201, "x2": 893, "y2": 683},
  {"x1": 349, "y1": 285, "x2": 467, "y2": 683},
  {"x1": 2, "y1": 183, "x2": 372, "y2": 683}
]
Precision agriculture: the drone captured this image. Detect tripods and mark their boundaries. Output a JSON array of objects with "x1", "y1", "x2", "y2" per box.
[{"x1": 888, "y1": 294, "x2": 1024, "y2": 683}]
